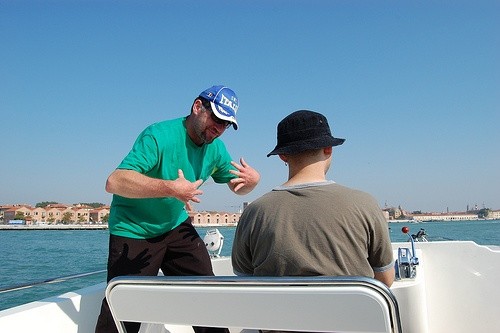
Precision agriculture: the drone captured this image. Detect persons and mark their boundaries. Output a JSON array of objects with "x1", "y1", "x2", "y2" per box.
[
  {"x1": 232, "y1": 110, "x2": 395, "y2": 333},
  {"x1": 94, "y1": 84, "x2": 260, "y2": 333}
]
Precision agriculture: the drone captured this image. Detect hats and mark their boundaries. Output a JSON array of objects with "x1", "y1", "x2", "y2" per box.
[
  {"x1": 199, "y1": 85, "x2": 239, "y2": 130},
  {"x1": 266, "y1": 110, "x2": 346, "y2": 157}
]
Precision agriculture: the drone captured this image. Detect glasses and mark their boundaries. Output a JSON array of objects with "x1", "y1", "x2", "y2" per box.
[{"x1": 208, "y1": 109, "x2": 229, "y2": 126}]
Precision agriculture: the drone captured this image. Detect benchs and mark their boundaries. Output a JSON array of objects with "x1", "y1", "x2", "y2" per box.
[{"x1": 105, "y1": 276, "x2": 402, "y2": 333}]
